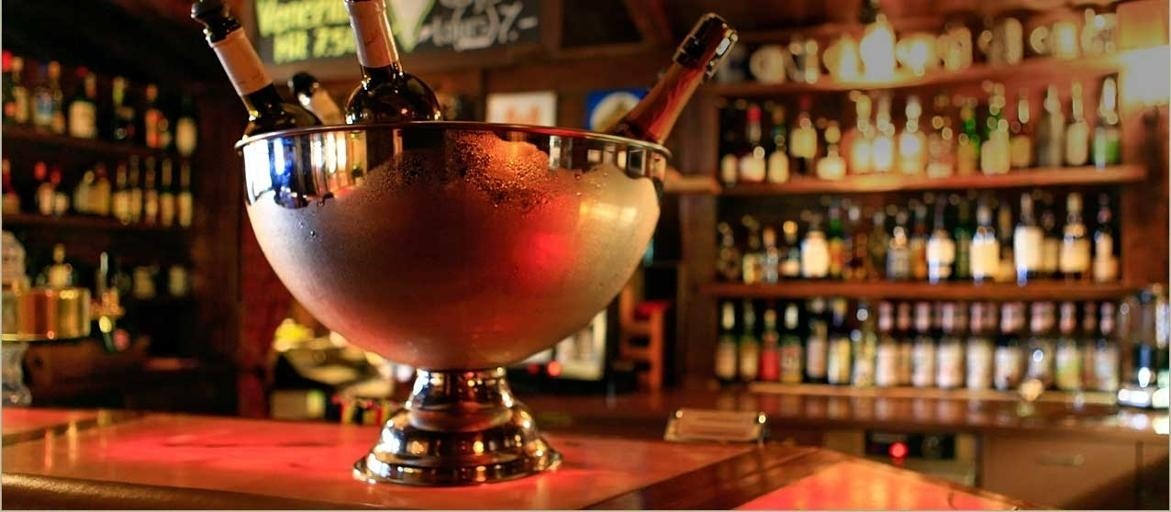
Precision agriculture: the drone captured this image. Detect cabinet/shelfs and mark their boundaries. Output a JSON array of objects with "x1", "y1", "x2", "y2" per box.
[
  {"x1": 978, "y1": 437, "x2": 1169, "y2": 510},
  {"x1": 2, "y1": 46, "x2": 245, "y2": 416},
  {"x1": 698, "y1": 2, "x2": 1151, "y2": 413}
]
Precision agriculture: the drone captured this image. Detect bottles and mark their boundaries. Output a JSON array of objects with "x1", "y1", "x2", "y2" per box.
[
  {"x1": 184, "y1": 1, "x2": 320, "y2": 132},
  {"x1": 710, "y1": 281, "x2": 1171, "y2": 406},
  {"x1": 284, "y1": 66, "x2": 345, "y2": 125},
  {"x1": 343, "y1": 0, "x2": 438, "y2": 132},
  {"x1": 607, "y1": 10, "x2": 738, "y2": 138},
  {"x1": 712, "y1": 188, "x2": 1127, "y2": 288},
  {"x1": 47, "y1": 264, "x2": 196, "y2": 347},
  {"x1": 0, "y1": 158, "x2": 203, "y2": 224},
  {"x1": 0, "y1": 50, "x2": 201, "y2": 152},
  {"x1": 723, "y1": 83, "x2": 1135, "y2": 177}
]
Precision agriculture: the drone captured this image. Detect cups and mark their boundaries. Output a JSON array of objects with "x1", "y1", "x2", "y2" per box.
[{"x1": 746, "y1": 5, "x2": 1140, "y2": 78}]
